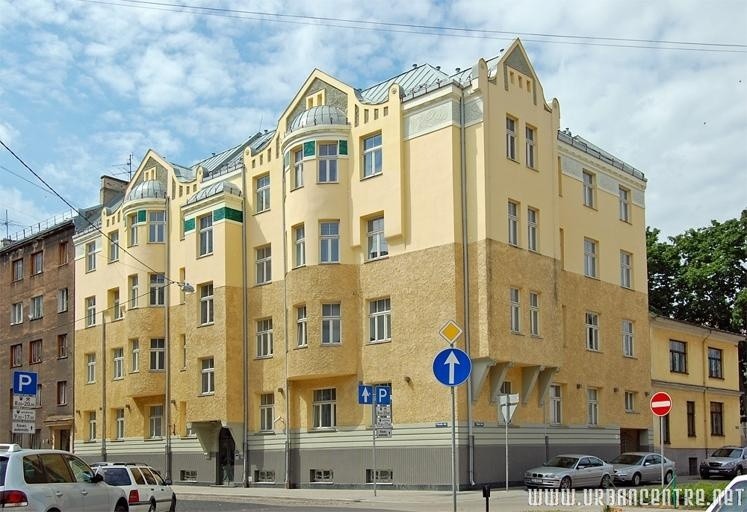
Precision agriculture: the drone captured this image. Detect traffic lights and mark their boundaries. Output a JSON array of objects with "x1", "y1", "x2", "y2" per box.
[{"x1": 11, "y1": 421, "x2": 36, "y2": 434}]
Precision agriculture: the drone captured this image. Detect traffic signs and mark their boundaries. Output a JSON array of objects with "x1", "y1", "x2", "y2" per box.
[
  {"x1": 12, "y1": 395, "x2": 37, "y2": 409},
  {"x1": 375, "y1": 407, "x2": 392, "y2": 417},
  {"x1": 375, "y1": 428, "x2": 393, "y2": 438},
  {"x1": 376, "y1": 417, "x2": 393, "y2": 428},
  {"x1": 11, "y1": 409, "x2": 37, "y2": 422}
]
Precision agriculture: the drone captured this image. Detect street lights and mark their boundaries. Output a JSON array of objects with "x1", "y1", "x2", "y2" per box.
[
  {"x1": 164, "y1": 278, "x2": 196, "y2": 486},
  {"x1": 539, "y1": 400, "x2": 550, "y2": 460}
]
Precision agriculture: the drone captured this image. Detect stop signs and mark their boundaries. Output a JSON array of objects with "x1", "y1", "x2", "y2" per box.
[{"x1": 649, "y1": 391, "x2": 674, "y2": 418}]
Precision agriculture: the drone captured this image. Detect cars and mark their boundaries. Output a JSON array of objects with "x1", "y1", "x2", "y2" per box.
[
  {"x1": 608, "y1": 451, "x2": 677, "y2": 488},
  {"x1": 86, "y1": 461, "x2": 178, "y2": 512},
  {"x1": 521, "y1": 452, "x2": 617, "y2": 493},
  {"x1": 704, "y1": 474, "x2": 747, "y2": 512}
]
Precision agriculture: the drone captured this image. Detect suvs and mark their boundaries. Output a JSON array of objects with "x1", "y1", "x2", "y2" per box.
[
  {"x1": 0, "y1": 442, "x2": 131, "y2": 512},
  {"x1": 698, "y1": 443, "x2": 747, "y2": 480}
]
